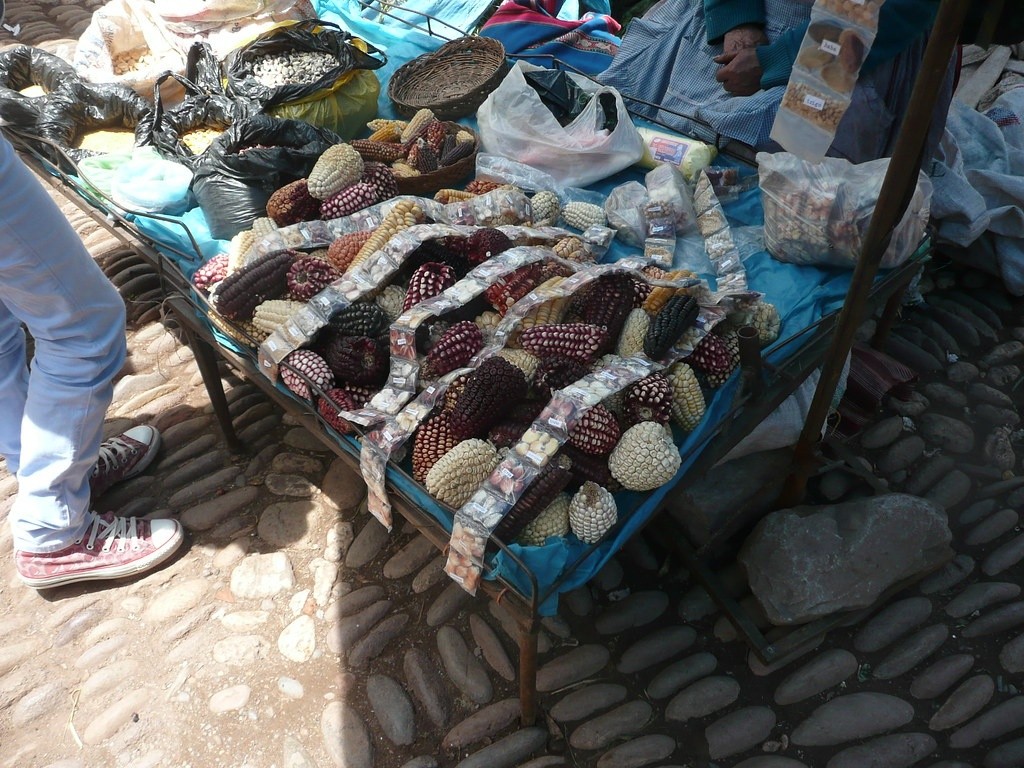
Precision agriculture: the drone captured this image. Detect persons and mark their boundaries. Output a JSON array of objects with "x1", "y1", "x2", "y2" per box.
[
  {"x1": 598, "y1": 0, "x2": 963, "y2": 176},
  {"x1": 0, "y1": 133, "x2": 185, "y2": 589}
]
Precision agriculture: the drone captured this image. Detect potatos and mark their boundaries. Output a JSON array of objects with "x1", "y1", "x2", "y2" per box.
[{"x1": 2, "y1": 0, "x2": 110, "y2": 42}]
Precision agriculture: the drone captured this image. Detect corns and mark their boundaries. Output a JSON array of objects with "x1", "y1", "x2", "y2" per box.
[{"x1": 190, "y1": 107, "x2": 781, "y2": 547}]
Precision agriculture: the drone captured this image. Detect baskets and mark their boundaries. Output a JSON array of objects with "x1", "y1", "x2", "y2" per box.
[
  {"x1": 393, "y1": 121, "x2": 479, "y2": 196},
  {"x1": 389, "y1": 37, "x2": 506, "y2": 121}
]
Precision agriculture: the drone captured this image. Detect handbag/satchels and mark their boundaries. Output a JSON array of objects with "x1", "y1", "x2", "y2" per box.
[{"x1": 478, "y1": 60, "x2": 644, "y2": 186}]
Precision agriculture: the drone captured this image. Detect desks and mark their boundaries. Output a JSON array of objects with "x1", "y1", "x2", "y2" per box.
[{"x1": 0, "y1": 0, "x2": 941, "y2": 725}]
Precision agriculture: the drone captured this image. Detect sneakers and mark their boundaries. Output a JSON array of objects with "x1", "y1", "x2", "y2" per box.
[
  {"x1": 88, "y1": 425, "x2": 160, "y2": 509},
  {"x1": 16, "y1": 511, "x2": 185, "y2": 590}
]
